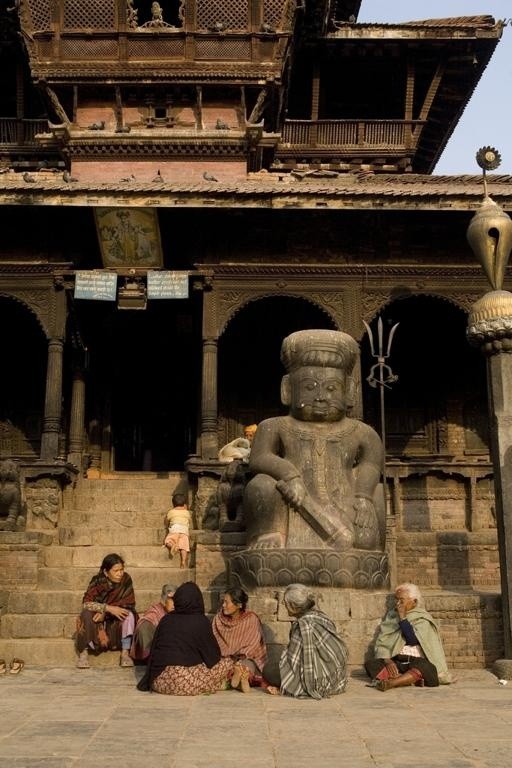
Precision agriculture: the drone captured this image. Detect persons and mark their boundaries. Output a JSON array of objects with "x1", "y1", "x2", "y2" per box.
[
  {"x1": 209, "y1": 586, "x2": 268, "y2": 693},
  {"x1": 163, "y1": 493, "x2": 193, "y2": 569},
  {"x1": 74, "y1": 553, "x2": 140, "y2": 668},
  {"x1": 244, "y1": 425, "x2": 257, "y2": 447},
  {"x1": 136, "y1": 582, "x2": 235, "y2": 696},
  {"x1": 246, "y1": 330, "x2": 383, "y2": 552},
  {"x1": 264, "y1": 582, "x2": 350, "y2": 702},
  {"x1": 129, "y1": 584, "x2": 177, "y2": 663},
  {"x1": 366, "y1": 583, "x2": 457, "y2": 691}
]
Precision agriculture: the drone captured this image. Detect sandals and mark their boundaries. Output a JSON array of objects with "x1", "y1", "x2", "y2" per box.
[
  {"x1": 0, "y1": 657, "x2": 24, "y2": 676},
  {"x1": 76, "y1": 655, "x2": 90, "y2": 668},
  {"x1": 119, "y1": 654, "x2": 132, "y2": 668}
]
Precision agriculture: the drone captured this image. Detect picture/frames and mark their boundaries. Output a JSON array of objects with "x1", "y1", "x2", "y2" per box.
[{"x1": 93, "y1": 206, "x2": 164, "y2": 269}]
